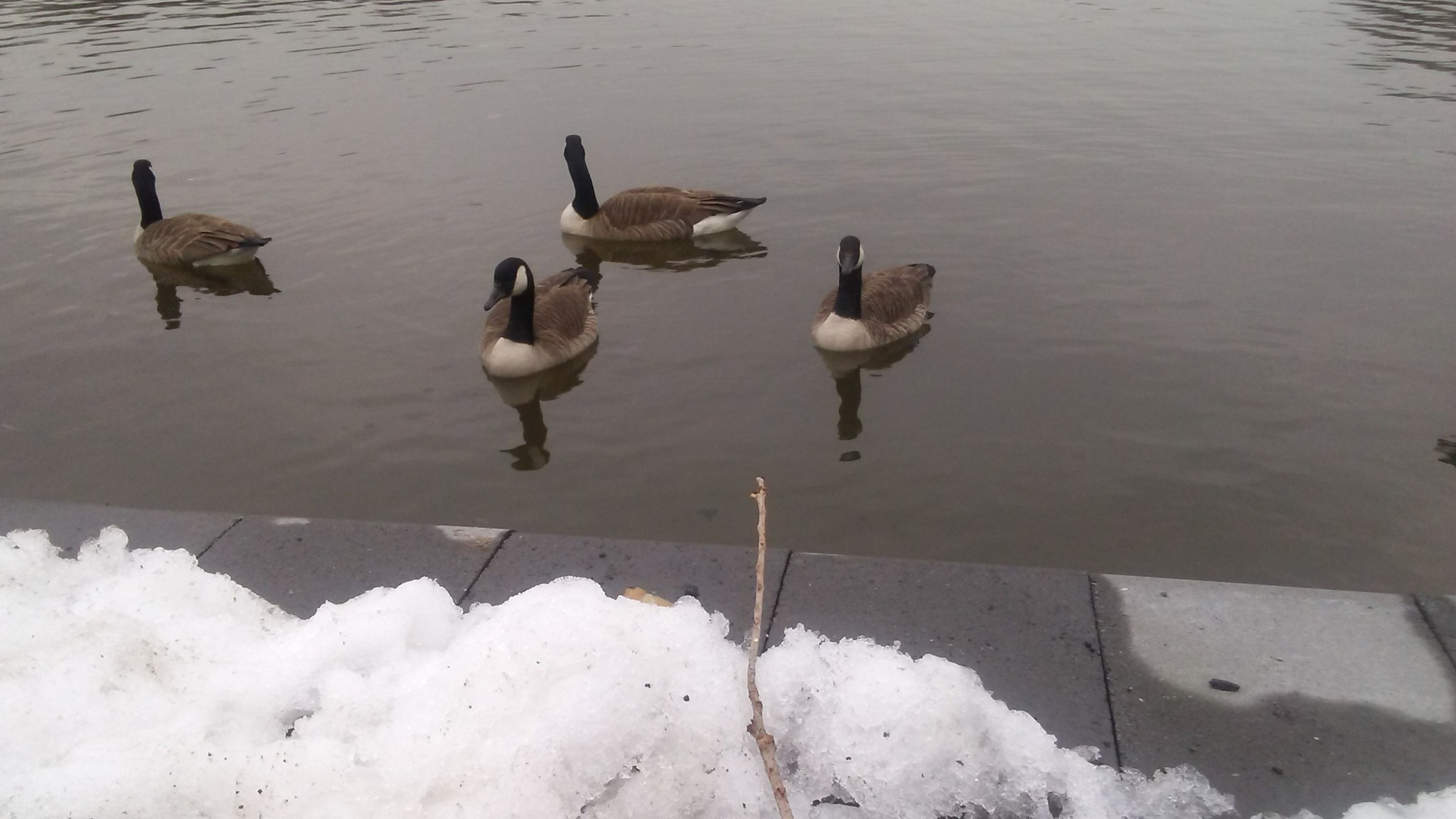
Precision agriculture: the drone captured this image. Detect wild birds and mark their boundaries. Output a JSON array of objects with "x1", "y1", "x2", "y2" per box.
[
  {"x1": 561, "y1": 228, "x2": 769, "y2": 295},
  {"x1": 560, "y1": 135, "x2": 767, "y2": 241},
  {"x1": 132, "y1": 159, "x2": 272, "y2": 267},
  {"x1": 482, "y1": 334, "x2": 599, "y2": 471},
  {"x1": 479, "y1": 257, "x2": 599, "y2": 378},
  {"x1": 138, "y1": 257, "x2": 282, "y2": 330},
  {"x1": 814, "y1": 310, "x2": 935, "y2": 461},
  {"x1": 810, "y1": 236, "x2": 939, "y2": 352}
]
